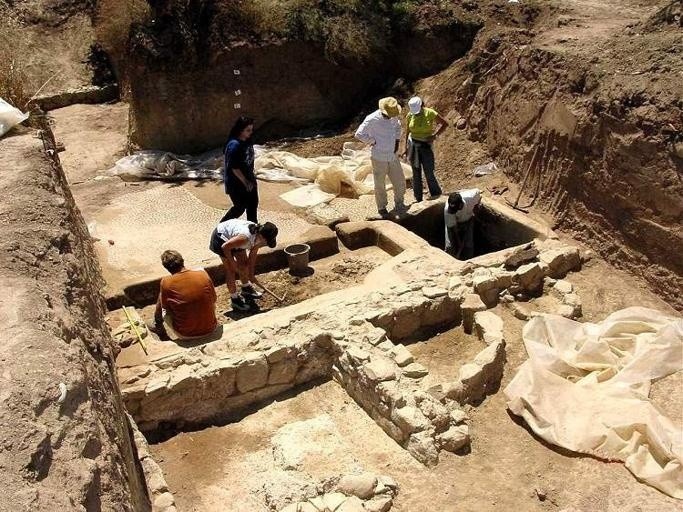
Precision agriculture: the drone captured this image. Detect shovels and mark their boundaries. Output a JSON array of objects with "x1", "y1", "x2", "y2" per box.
[{"x1": 109, "y1": 296, "x2": 149, "y2": 355}]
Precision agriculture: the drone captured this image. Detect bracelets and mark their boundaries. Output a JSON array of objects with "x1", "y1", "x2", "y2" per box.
[{"x1": 435, "y1": 133, "x2": 438, "y2": 137}]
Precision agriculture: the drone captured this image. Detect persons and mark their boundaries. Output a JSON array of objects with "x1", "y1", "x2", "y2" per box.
[
  {"x1": 442, "y1": 188, "x2": 483, "y2": 261},
  {"x1": 354, "y1": 95, "x2": 407, "y2": 219},
  {"x1": 209, "y1": 218, "x2": 278, "y2": 311},
  {"x1": 152, "y1": 249, "x2": 218, "y2": 341},
  {"x1": 218, "y1": 116, "x2": 258, "y2": 224},
  {"x1": 403, "y1": 96, "x2": 449, "y2": 202}
]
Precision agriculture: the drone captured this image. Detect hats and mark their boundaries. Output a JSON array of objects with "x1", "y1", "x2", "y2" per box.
[
  {"x1": 408, "y1": 97, "x2": 421, "y2": 115},
  {"x1": 447, "y1": 193, "x2": 462, "y2": 215},
  {"x1": 259, "y1": 222, "x2": 278, "y2": 248},
  {"x1": 378, "y1": 97, "x2": 402, "y2": 118}
]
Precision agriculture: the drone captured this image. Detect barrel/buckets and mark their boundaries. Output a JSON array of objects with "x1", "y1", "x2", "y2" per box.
[{"x1": 284, "y1": 243, "x2": 311, "y2": 273}]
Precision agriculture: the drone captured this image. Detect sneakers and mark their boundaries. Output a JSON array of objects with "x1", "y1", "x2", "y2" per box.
[
  {"x1": 240, "y1": 284, "x2": 262, "y2": 299},
  {"x1": 230, "y1": 296, "x2": 251, "y2": 311}
]
